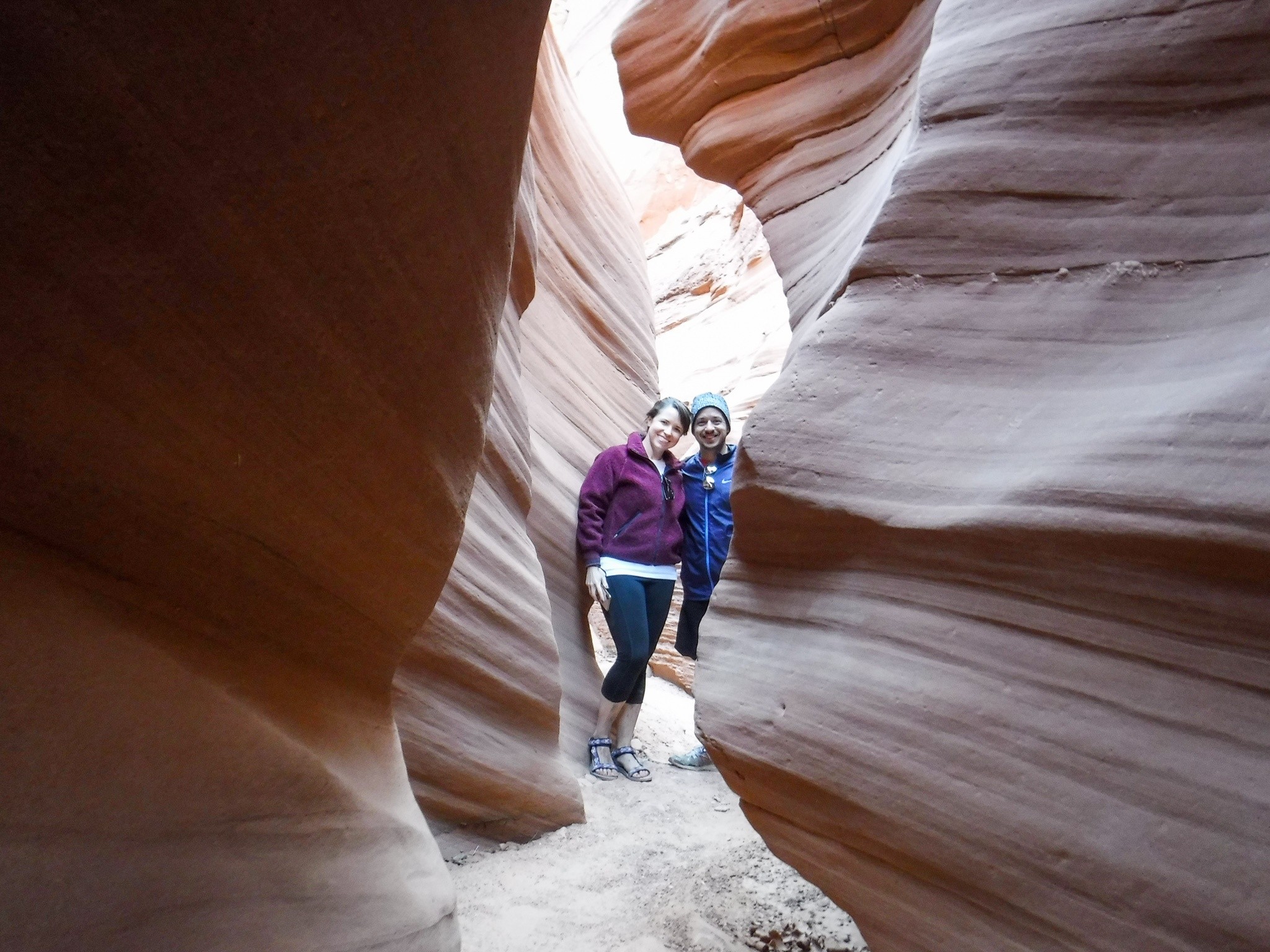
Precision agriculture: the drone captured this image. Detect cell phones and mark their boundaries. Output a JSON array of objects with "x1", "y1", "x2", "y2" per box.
[{"x1": 595, "y1": 584, "x2": 611, "y2": 612}]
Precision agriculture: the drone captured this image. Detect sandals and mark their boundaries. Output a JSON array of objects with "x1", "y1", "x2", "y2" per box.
[
  {"x1": 610, "y1": 746, "x2": 652, "y2": 781},
  {"x1": 586, "y1": 736, "x2": 619, "y2": 781}
]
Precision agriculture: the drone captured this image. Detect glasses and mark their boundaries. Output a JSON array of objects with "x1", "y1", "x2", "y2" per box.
[
  {"x1": 703, "y1": 462, "x2": 718, "y2": 490},
  {"x1": 660, "y1": 474, "x2": 675, "y2": 501}
]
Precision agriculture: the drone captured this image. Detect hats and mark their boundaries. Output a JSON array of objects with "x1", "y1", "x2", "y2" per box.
[{"x1": 690, "y1": 392, "x2": 731, "y2": 436}]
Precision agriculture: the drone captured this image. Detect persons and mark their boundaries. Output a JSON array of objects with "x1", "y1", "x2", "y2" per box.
[
  {"x1": 576, "y1": 397, "x2": 695, "y2": 784},
  {"x1": 668, "y1": 391, "x2": 737, "y2": 771}
]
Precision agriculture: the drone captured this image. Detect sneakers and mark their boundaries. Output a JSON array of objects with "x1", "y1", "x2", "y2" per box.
[{"x1": 669, "y1": 746, "x2": 719, "y2": 772}]
